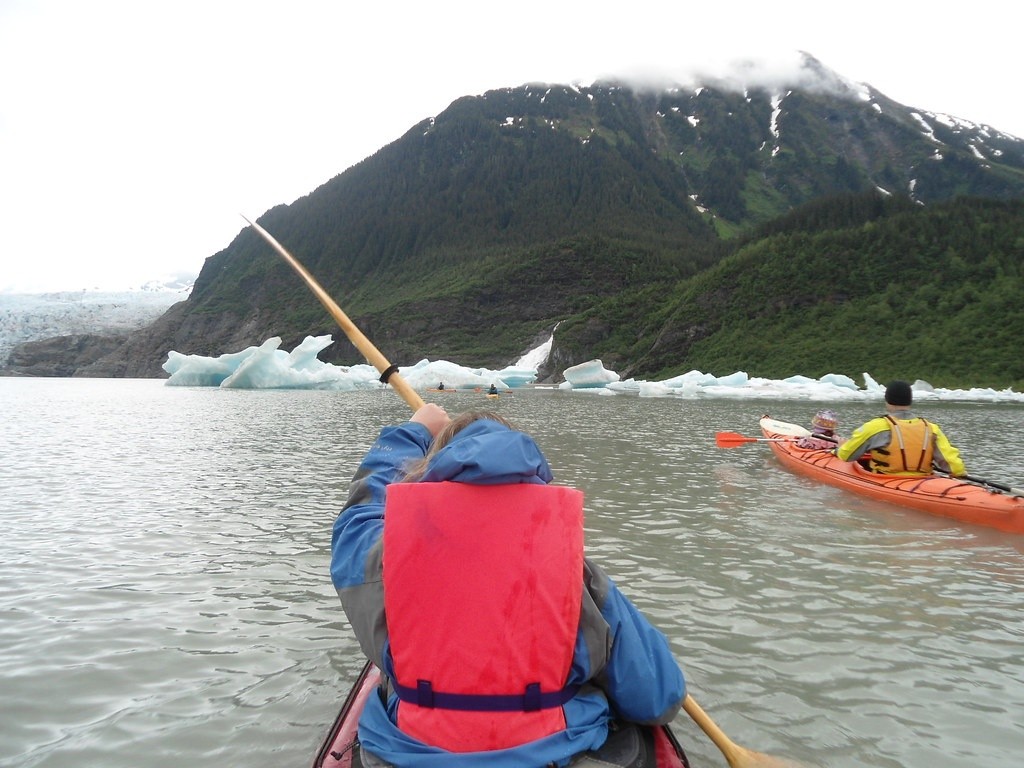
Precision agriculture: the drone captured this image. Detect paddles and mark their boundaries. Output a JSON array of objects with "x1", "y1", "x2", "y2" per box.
[
  {"x1": 474, "y1": 388, "x2": 513, "y2": 393},
  {"x1": 236, "y1": 208, "x2": 773, "y2": 767},
  {"x1": 811, "y1": 433, "x2": 1024, "y2": 497},
  {"x1": 715, "y1": 431, "x2": 800, "y2": 449}
]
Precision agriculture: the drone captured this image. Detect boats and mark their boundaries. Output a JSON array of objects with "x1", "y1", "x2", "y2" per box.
[
  {"x1": 760, "y1": 412, "x2": 1024, "y2": 536},
  {"x1": 425, "y1": 387, "x2": 456, "y2": 393},
  {"x1": 312, "y1": 658, "x2": 690, "y2": 768},
  {"x1": 486, "y1": 393, "x2": 499, "y2": 399}
]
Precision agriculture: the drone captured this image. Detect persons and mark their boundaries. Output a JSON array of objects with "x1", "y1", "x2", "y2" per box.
[
  {"x1": 438, "y1": 382, "x2": 444, "y2": 390},
  {"x1": 330, "y1": 403, "x2": 687, "y2": 768},
  {"x1": 793, "y1": 409, "x2": 840, "y2": 449},
  {"x1": 488, "y1": 384, "x2": 497, "y2": 394},
  {"x1": 831, "y1": 380, "x2": 968, "y2": 476}
]
